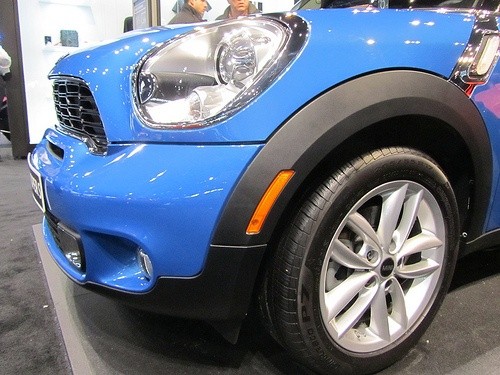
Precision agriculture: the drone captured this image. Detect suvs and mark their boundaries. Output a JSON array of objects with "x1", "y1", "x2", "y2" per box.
[{"x1": 26, "y1": 0, "x2": 500, "y2": 375}]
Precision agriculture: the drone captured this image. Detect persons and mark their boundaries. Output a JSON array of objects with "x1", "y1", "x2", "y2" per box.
[
  {"x1": 216, "y1": 0, "x2": 261, "y2": 21},
  {"x1": 168, "y1": 0, "x2": 208, "y2": 25}
]
